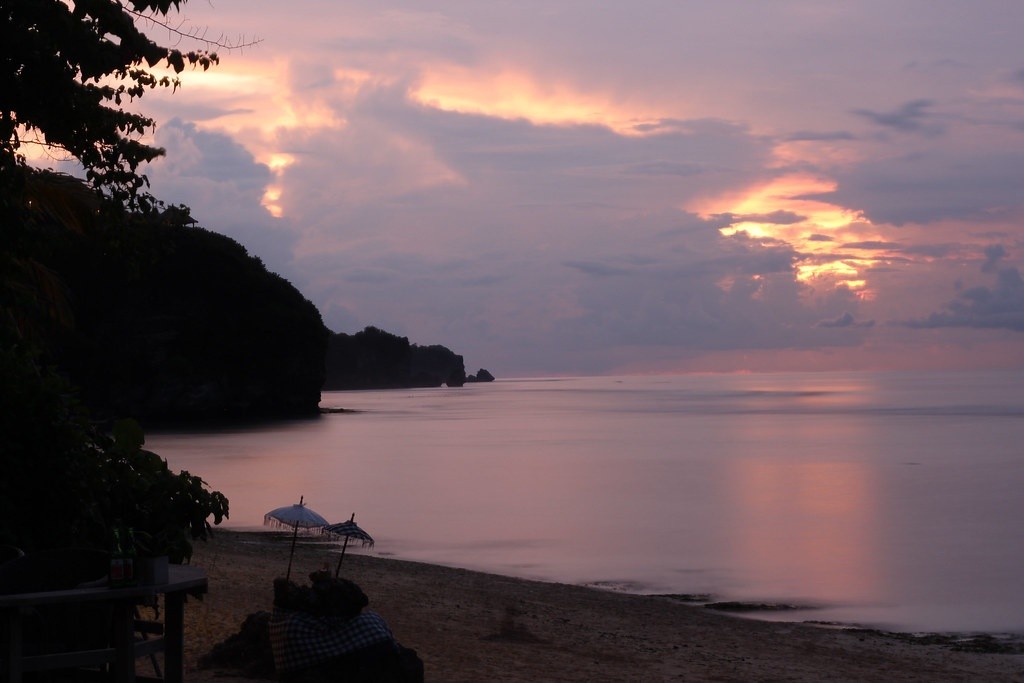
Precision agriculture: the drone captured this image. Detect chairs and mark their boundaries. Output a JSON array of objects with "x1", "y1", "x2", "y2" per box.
[{"x1": 0, "y1": 543, "x2": 134, "y2": 683}]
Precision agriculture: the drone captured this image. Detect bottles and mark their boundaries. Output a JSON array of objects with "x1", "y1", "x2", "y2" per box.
[
  {"x1": 124, "y1": 527, "x2": 137, "y2": 587},
  {"x1": 110, "y1": 528, "x2": 125, "y2": 588}
]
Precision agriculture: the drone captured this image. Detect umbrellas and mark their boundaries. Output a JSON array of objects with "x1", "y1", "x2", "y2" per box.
[
  {"x1": 320, "y1": 513, "x2": 373, "y2": 578},
  {"x1": 264, "y1": 497, "x2": 329, "y2": 582}
]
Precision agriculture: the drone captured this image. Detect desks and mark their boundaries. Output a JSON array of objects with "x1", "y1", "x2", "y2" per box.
[{"x1": 0, "y1": 578, "x2": 209, "y2": 683}]
